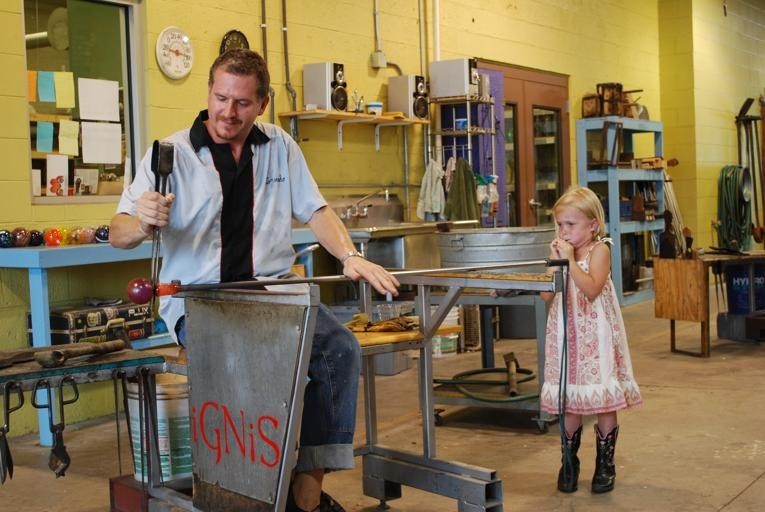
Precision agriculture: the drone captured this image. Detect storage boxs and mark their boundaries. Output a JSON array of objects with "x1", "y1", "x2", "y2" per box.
[
  {"x1": 327, "y1": 299, "x2": 417, "y2": 376},
  {"x1": 25, "y1": 301, "x2": 152, "y2": 347}
]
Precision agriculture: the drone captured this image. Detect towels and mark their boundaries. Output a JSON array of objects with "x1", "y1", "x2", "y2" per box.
[{"x1": 415, "y1": 156, "x2": 481, "y2": 230}]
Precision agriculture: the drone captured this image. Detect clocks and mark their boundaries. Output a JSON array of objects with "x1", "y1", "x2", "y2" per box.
[
  {"x1": 219, "y1": 29, "x2": 250, "y2": 55},
  {"x1": 155, "y1": 25, "x2": 194, "y2": 81}
]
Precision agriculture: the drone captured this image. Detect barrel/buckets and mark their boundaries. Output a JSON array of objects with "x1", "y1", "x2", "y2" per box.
[
  {"x1": 125, "y1": 372, "x2": 194, "y2": 490},
  {"x1": 429, "y1": 305, "x2": 459, "y2": 356}
]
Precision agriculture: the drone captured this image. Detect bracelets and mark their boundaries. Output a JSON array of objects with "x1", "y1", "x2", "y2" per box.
[{"x1": 338, "y1": 251, "x2": 366, "y2": 264}]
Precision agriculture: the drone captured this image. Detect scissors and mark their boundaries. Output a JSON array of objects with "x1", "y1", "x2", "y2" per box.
[{"x1": 0, "y1": 382, "x2": 24, "y2": 484}]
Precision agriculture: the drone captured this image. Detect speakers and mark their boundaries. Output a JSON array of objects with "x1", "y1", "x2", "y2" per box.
[
  {"x1": 302, "y1": 62, "x2": 348, "y2": 112},
  {"x1": 387, "y1": 74, "x2": 428, "y2": 120}
]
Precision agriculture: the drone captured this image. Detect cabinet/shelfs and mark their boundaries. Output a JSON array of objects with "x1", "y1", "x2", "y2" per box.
[
  {"x1": 575, "y1": 117, "x2": 667, "y2": 308},
  {"x1": 415, "y1": 290, "x2": 564, "y2": 435},
  {"x1": 428, "y1": 93, "x2": 498, "y2": 228}
]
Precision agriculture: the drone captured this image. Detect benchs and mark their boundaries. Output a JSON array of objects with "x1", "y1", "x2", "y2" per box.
[{"x1": 144, "y1": 322, "x2": 502, "y2": 512}]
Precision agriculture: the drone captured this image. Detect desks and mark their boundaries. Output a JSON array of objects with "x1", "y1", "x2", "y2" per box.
[
  {"x1": 652, "y1": 249, "x2": 765, "y2": 357},
  {"x1": 0, "y1": 226, "x2": 374, "y2": 448}
]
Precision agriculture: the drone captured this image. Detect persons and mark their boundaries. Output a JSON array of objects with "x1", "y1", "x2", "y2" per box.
[
  {"x1": 537, "y1": 185, "x2": 644, "y2": 494},
  {"x1": 108, "y1": 46, "x2": 404, "y2": 512}
]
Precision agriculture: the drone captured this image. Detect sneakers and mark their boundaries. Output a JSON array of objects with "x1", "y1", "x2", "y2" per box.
[{"x1": 287, "y1": 484, "x2": 345, "y2": 512}]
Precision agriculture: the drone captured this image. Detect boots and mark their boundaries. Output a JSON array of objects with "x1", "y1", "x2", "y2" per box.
[
  {"x1": 591, "y1": 425, "x2": 619, "y2": 493},
  {"x1": 558, "y1": 424, "x2": 582, "y2": 492}
]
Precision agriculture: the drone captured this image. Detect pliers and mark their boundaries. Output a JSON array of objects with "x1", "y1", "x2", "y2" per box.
[{"x1": 30, "y1": 376, "x2": 79, "y2": 478}]
